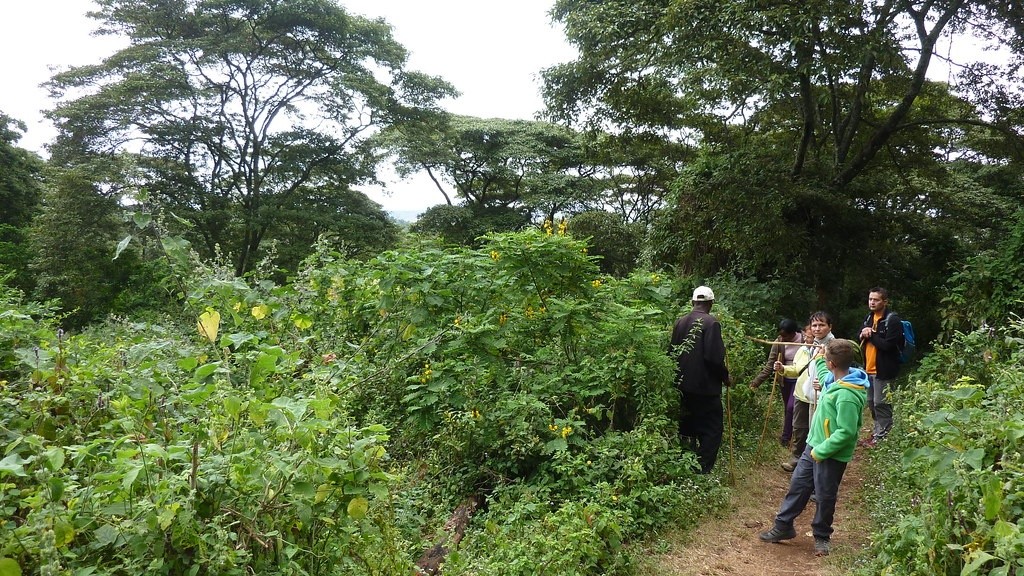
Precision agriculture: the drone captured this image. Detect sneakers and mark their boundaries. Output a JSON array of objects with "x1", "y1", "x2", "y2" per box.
[
  {"x1": 815, "y1": 537, "x2": 829, "y2": 556},
  {"x1": 760, "y1": 526, "x2": 796, "y2": 542}
]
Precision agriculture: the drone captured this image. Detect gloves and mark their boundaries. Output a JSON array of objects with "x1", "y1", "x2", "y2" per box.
[{"x1": 723, "y1": 374, "x2": 733, "y2": 386}]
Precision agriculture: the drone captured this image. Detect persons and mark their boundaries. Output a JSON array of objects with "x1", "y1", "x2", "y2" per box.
[
  {"x1": 668, "y1": 287, "x2": 730, "y2": 476},
  {"x1": 749, "y1": 287, "x2": 905, "y2": 555}
]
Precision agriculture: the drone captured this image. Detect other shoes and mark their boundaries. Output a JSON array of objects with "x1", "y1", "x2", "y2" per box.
[
  {"x1": 810, "y1": 494, "x2": 816, "y2": 502},
  {"x1": 857, "y1": 434, "x2": 882, "y2": 449},
  {"x1": 781, "y1": 462, "x2": 795, "y2": 472}
]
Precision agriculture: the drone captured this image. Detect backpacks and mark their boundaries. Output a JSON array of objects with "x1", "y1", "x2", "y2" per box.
[{"x1": 886, "y1": 312, "x2": 915, "y2": 364}]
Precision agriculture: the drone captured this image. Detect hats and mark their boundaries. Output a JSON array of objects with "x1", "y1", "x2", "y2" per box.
[{"x1": 692, "y1": 286, "x2": 714, "y2": 301}]
